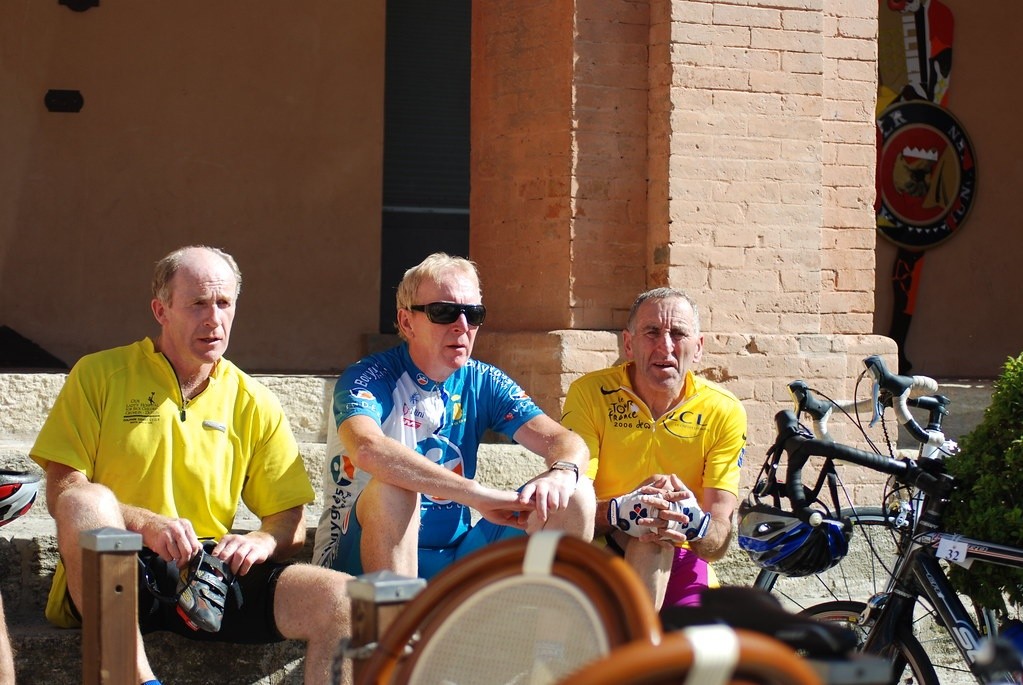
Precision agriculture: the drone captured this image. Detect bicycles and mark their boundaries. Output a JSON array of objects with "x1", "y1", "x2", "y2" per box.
[{"x1": 737, "y1": 352, "x2": 1023, "y2": 685}]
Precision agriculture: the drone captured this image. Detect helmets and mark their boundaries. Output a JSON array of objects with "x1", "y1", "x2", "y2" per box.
[
  {"x1": 737, "y1": 500, "x2": 853, "y2": 577},
  {"x1": 0, "y1": 472, "x2": 39, "y2": 527}
]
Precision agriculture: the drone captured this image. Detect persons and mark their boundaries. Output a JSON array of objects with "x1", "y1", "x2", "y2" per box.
[
  {"x1": 312, "y1": 251, "x2": 597, "y2": 651},
  {"x1": 559, "y1": 289, "x2": 749, "y2": 612},
  {"x1": 45, "y1": 246, "x2": 354, "y2": 685},
  {"x1": 1, "y1": 589, "x2": 18, "y2": 685}
]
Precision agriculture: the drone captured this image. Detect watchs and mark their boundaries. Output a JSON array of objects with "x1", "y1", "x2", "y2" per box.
[{"x1": 549, "y1": 460, "x2": 579, "y2": 483}]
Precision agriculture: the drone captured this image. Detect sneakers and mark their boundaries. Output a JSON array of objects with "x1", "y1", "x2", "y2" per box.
[{"x1": 178, "y1": 541, "x2": 233, "y2": 633}]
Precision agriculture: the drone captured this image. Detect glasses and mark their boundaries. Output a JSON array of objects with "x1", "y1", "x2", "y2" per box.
[{"x1": 405, "y1": 300, "x2": 487, "y2": 326}]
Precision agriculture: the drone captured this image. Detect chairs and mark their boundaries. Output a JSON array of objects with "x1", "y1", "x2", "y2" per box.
[
  {"x1": 558, "y1": 625, "x2": 822, "y2": 685},
  {"x1": 358, "y1": 530, "x2": 664, "y2": 685}
]
